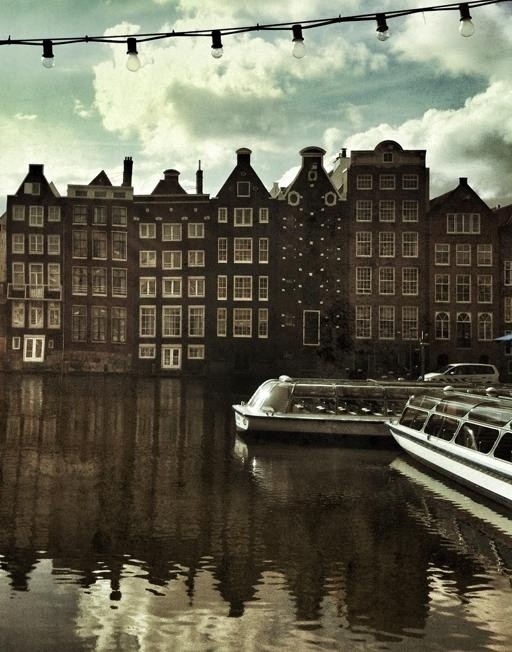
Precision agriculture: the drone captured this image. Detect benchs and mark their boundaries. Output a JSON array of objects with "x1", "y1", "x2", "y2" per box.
[{"x1": 292, "y1": 397, "x2": 406, "y2": 417}]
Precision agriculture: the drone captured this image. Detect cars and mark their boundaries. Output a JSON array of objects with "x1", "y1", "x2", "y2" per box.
[{"x1": 424, "y1": 362, "x2": 499, "y2": 383}]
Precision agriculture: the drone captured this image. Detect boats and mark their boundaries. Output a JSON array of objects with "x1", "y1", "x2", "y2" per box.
[
  {"x1": 389, "y1": 453, "x2": 512, "y2": 576},
  {"x1": 232, "y1": 375, "x2": 512, "y2": 509}
]
